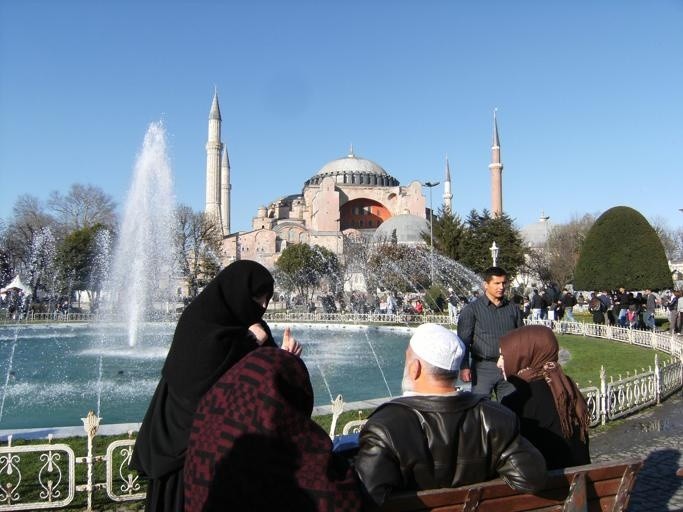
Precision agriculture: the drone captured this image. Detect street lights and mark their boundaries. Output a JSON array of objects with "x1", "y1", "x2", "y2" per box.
[
  {"x1": 489, "y1": 241, "x2": 499, "y2": 266},
  {"x1": 421, "y1": 182, "x2": 440, "y2": 284}
]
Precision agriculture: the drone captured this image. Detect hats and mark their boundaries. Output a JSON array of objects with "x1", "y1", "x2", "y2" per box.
[{"x1": 410, "y1": 322, "x2": 467, "y2": 371}]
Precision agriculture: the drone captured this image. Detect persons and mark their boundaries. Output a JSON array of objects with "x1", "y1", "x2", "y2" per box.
[
  {"x1": 55, "y1": 301, "x2": 62, "y2": 319},
  {"x1": 355, "y1": 321, "x2": 548, "y2": 512},
  {"x1": 184, "y1": 346, "x2": 380, "y2": 511},
  {"x1": 457, "y1": 266, "x2": 526, "y2": 400},
  {"x1": 62, "y1": 300, "x2": 72, "y2": 320},
  {"x1": 127, "y1": 259, "x2": 304, "y2": 510},
  {"x1": 489, "y1": 324, "x2": 591, "y2": 475}
]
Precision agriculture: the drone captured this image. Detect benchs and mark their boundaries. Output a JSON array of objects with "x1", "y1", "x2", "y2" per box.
[{"x1": 394, "y1": 459, "x2": 645, "y2": 510}]
